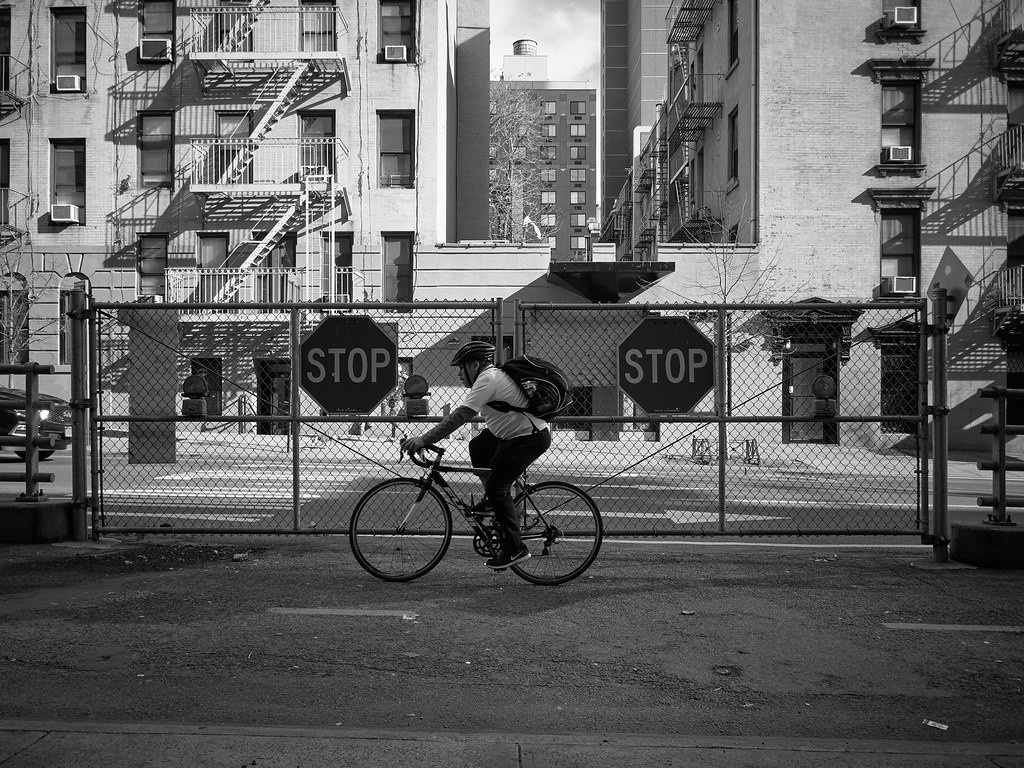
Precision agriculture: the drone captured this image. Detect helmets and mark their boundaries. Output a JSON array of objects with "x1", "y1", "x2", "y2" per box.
[{"x1": 450, "y1": 341, "x2": 496, "y2": 366}]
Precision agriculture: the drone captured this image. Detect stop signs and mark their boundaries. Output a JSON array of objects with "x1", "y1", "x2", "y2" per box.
[
  {"x1": 299, "y1": 314, "x2": 399, "y2": 415},
  {"x1": 618, "y1": 317, "x2": 715, "y2": 416}
]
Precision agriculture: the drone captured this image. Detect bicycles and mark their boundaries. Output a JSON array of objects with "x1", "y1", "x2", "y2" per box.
[{"x1": 349, "y1": 433, "x2": 604, "y2": 585}]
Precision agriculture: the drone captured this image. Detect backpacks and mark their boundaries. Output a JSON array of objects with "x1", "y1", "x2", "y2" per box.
[{"x1": 481, "y1": 356, "x2": 573, "y2": 420}]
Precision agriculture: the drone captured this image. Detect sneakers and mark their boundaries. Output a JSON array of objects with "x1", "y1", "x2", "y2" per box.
[
  {"x1": 486, "y1": 542, "x2": 532, "y2": 569},
  {"x1": 471, "y1": 500, "x2": 495, "y2": 516}
]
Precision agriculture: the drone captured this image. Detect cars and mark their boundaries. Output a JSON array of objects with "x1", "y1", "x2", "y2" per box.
[{"x1": 0, "y1": 384, "x2": 73, "y2": 461}]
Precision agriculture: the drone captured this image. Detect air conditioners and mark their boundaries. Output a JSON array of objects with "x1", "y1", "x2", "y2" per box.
[
  {"x1": 50, "y1": 204, "x2": 80, "y2": 222},
  {"x1": 888, "y1": 7, "x2": 917, "y2": 27},
  {"x1": 56, "y1": 75, "x2": 82, "y2": 91},
  {"x1": 322, "y1": 294, "x2": 350, "y2": 311},
  {"x1": 385, "y1": 45, "x2": 407, "y2": 63},
  {"x1": 139, "y1": 38, "x2": 173, "y2": 62},
  {"x1": 301, "y1": 166, "x2": 330, "y2": 183},
  {"x1": 888, "y1": 275, "x2": 917, "y2": 293},
  {"x1": 885, "y1": 146, "x2": 912, "y2": 162},
  {"x1": 137, "y1": 295, "x2": 163, "y2": 303}
]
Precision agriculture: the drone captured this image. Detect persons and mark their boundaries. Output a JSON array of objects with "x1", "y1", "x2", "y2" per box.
[{"x1": 400, "y1": 341, "x2": 552, "y2": 568}]
